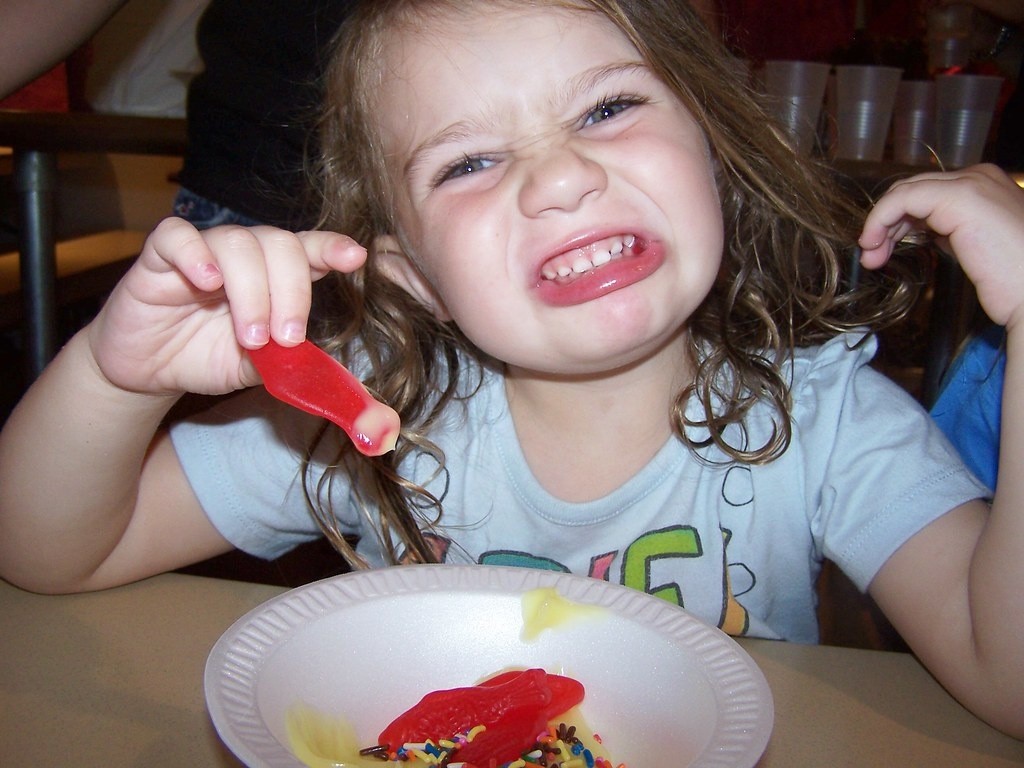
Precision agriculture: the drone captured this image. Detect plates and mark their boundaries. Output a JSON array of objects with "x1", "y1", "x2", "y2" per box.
[{"x1": 204, "y1": 563, "x2": 775, "y2": 768}]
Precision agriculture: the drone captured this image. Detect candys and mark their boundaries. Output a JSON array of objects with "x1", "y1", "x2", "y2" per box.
[{"x1": 250, "y1": 339, "x2": 398, "y2": 454}]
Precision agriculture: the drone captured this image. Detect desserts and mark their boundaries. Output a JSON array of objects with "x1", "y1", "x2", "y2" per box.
[{"x1": 357, "y1": 667, "x2": 615, "y2": 768}]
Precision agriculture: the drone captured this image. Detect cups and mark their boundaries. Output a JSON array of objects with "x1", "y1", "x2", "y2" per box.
[
  {"x1": 835, "y1": 65, "x2": 902, "y2": 161},
  {"x1": 894, "y1": 80, "x2": 938, "y2": 166},
  {"x1": 934, "y1": 76, "x2": 1003, "y2": 168},
  {"x1": 826, "y1": 77, "x2": 839, "y2": 159},
  {"x1": 766, "y1": 60, "x2": 830, "y2": 157}
]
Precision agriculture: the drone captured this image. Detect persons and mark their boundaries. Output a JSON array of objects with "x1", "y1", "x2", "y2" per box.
[{"x1": 1, "y1": 1, "x2": 1024, "y2": 744}]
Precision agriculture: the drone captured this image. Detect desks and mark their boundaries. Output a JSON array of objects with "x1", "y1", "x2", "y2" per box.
[{"x1": 0, "y1": 571, "x2": 1024, "y2": 768}]
